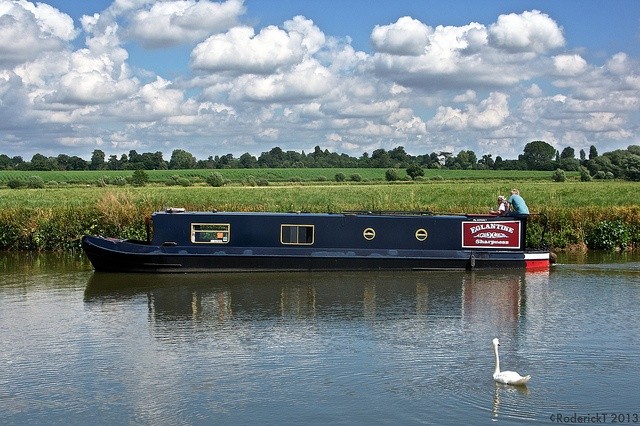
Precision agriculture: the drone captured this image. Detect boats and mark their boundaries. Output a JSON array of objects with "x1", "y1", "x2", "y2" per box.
[{"x1": 81, "y1": 206, "x2": 556, "y2": 274}]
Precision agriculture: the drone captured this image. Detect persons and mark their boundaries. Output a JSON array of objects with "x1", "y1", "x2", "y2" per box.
[
  {"x1": 489, "y1": 195, "x2": 512, "y2": 215},
  {"x1": 506, "y1": 189, "x2": 529, "y2": 217}
]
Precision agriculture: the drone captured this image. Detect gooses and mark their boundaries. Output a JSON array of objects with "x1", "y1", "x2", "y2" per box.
[{"x1": 493, "y1": 338, "x2": 531, "y2": 385}]
